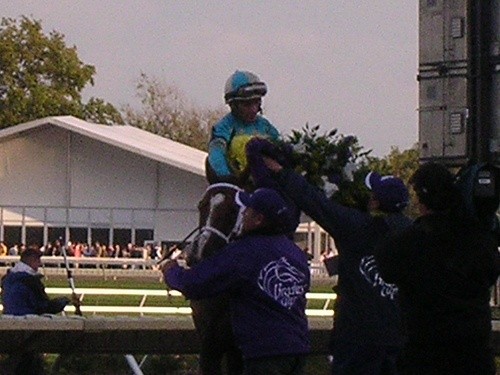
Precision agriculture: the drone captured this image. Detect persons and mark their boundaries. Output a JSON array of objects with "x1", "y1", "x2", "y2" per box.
[
  {"x1": 206, "y1": 69, "x2": 280, "y2": 195},
  {"x1": 0, "y1": 248, "x2": 71, "y2": 375},
  {"x1": 159, "y1": 187, "x2": 310, "y2": 375},
  {"x1": 374, "y1": 161, "x2": 500, "y2": 375},
  {"x1": 0, "y1": 237, "x2": 182, "y2": 270},
  {"x1": 246, "y1": 138, "x2": 413, "y2": 375}
]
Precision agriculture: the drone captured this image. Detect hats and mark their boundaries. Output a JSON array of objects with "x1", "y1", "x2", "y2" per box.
[
  {"x1": 234, "y1": 187, "x2": 289, "y2": 226},
  {"x1": 21, "y1": 249, "x2": 42, "y2": 263},
  {"x1": 365, "y1": 170, "x2": 409, "y2": 212}
]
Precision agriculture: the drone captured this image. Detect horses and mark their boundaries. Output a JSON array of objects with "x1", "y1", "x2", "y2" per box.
[{"x1": 186, "y1": 157, "x2": 258, "y2": 375}]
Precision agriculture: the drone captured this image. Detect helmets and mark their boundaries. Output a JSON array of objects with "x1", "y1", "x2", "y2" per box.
[{"x1": 224, "y1": 70, "x2": 267, "y2": 103}]
